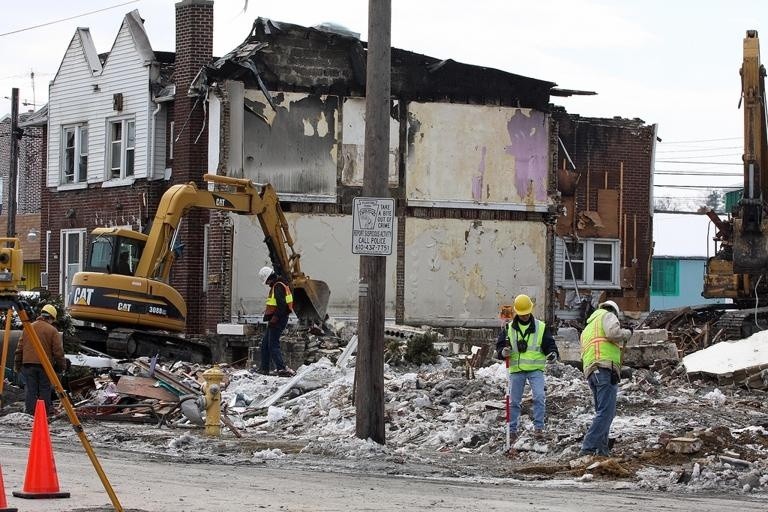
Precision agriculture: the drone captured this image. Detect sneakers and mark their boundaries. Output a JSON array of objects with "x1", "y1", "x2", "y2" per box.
[
  {"x1": 510, "y1": 433, "x2": 517, "y2": 444},
  {"x1": 535, "y1": 429, "x2": 544, "y2": 439}
]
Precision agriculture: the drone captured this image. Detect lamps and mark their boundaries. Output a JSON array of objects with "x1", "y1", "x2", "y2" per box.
[{"x1": 27, "y1": 227, "x2": 41, "y2": 242}]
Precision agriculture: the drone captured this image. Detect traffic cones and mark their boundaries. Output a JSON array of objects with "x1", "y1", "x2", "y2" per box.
[{"x1": 12, "y1": 398, "x2": 71, "y2": 499}]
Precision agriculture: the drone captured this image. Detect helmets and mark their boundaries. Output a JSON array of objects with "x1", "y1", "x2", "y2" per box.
[
  {"x1": 41, "y1": 303, "x2": 57, "y2": 320},
  {"x1": 599, "y1": 300, "x2": 620, "y2": 318},
  {"x1": 258, "y1": 266, "x2": 276, "y2": 285},
  {"x1": 513, "y1": 294, "x2": 533, "y2": 316}
]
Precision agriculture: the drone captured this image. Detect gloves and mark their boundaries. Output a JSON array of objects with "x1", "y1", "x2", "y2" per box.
[
  {"x1": 546, "y1": 352, "x2": 558, "y2": 364},
  {"x1": 501, "y1": 346, "x2": 511, "y2": 358}
]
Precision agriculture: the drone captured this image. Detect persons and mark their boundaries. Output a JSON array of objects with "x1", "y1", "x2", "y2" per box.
[
  {"x1": 248, "y1": 267, "x2": 294, "y2": 376},
  {"x1": 116, "y1": 249, "x2": 133, "y2": 275},
  {"x1": 495, "y1": 294, "x2": 559, "y2": 445},
  {"x1": 13, "y1": 302, "x2": 67, "y2": 425},
  {"x1": 578, "y1": 300, "x2": 634, "y2": 456}
]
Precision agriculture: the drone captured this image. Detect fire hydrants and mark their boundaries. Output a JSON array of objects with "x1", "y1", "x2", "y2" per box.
[{"x1": 195, "y1": 363, "x2": 225, "y2": 438}]
